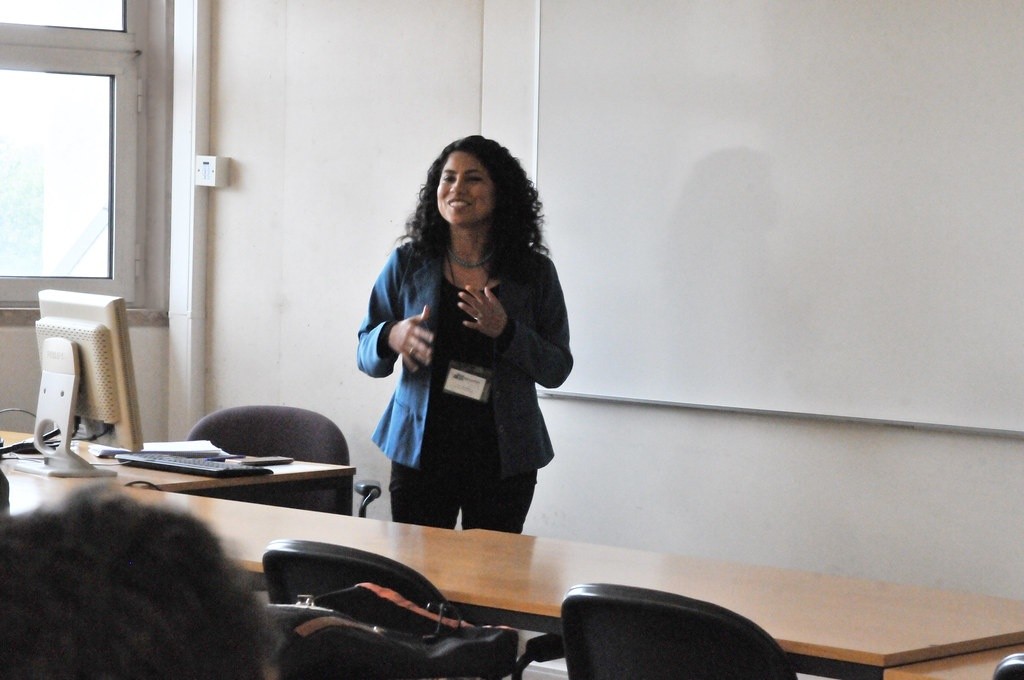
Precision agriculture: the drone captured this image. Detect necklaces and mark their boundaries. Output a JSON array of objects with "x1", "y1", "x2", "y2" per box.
[{"x1": 447, "y1": 246, "x2": 494, "y2": 267}]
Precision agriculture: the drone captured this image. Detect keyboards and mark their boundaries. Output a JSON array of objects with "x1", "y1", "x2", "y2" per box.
[{"x1": 115, "y1": 453, "x2": 274, "y2": 479}]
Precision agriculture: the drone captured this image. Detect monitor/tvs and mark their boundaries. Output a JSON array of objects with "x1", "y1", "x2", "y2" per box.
[{"x1": 12, "y1": 289, "x2": 144, "y2": 478}]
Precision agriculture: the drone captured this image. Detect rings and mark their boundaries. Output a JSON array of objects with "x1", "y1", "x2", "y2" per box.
[
  {"x1": 410, "y1": 348, "x2": 416, "y2": 356},
  {"x1": 474, "y1": 313, "x2": 481, "y2": 320}
]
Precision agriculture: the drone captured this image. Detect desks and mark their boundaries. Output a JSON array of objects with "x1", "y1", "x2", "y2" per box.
[
  {"x1": 0, "y1": 431, "x2": 356, "y2": 516},
  {"x1": 0, "y1": 468, "x2": 1024, "y2": 680}
]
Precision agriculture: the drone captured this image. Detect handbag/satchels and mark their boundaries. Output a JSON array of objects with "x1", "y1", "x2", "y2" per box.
[{"x1": 278, "y1": 580, "x2": 519, "y2": 680}]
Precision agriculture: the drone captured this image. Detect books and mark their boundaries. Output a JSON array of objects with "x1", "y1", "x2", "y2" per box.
[{"x1": 88, "y1": 439, "x2": 222, "y2": 457}]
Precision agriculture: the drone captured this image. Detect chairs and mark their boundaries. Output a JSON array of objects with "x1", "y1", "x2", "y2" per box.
[
  {"x1": 186, "y1": 406, "x2": 381, "y2": 518},
  {"x1": 261, "y1": 539, "x2": 799, "y2": 680}
]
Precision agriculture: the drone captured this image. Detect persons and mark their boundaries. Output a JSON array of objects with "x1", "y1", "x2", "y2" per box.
[
  {"x1": 0, "y1": 495, "x2": 271, "y2": 680},
  {"x1": 356, "y1": 136, "x2": 573, "y2": 535}
]
